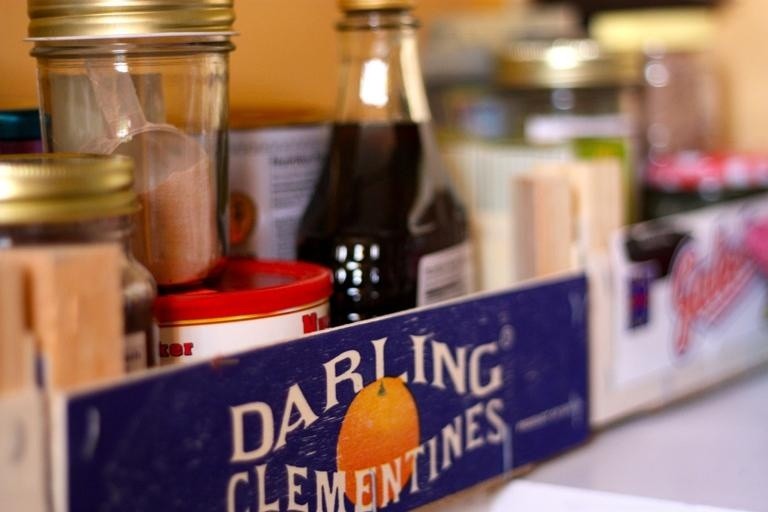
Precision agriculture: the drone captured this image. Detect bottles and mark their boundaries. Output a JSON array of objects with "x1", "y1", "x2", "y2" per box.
[
  {"x1": 495, "y1": 32, "x2": 645, "y2": 225},
  {"x1": 418, "y1": 0, "x2": 500, "y2": 145},
  {"x1": 592, "y1": 11, "x2": 725, "y2": 155},
  {"x1": 295, "y1": 0, "x2": 478, "y2": 322},
  {"x1": 25, "y1": 0, "x2": 238, "y2": 289},
  {"x1": 0, "y1": 151, "x2": 161, "y2": 373}
]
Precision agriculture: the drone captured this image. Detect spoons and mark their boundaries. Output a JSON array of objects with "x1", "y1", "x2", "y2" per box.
[{"x1": 88, "y1": 56, "x2": 210, "y2": 196}]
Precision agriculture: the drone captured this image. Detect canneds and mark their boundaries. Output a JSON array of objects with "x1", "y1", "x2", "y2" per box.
[{"x1": 228, "y1": 120, "x2": 336, "y2": 257}]
